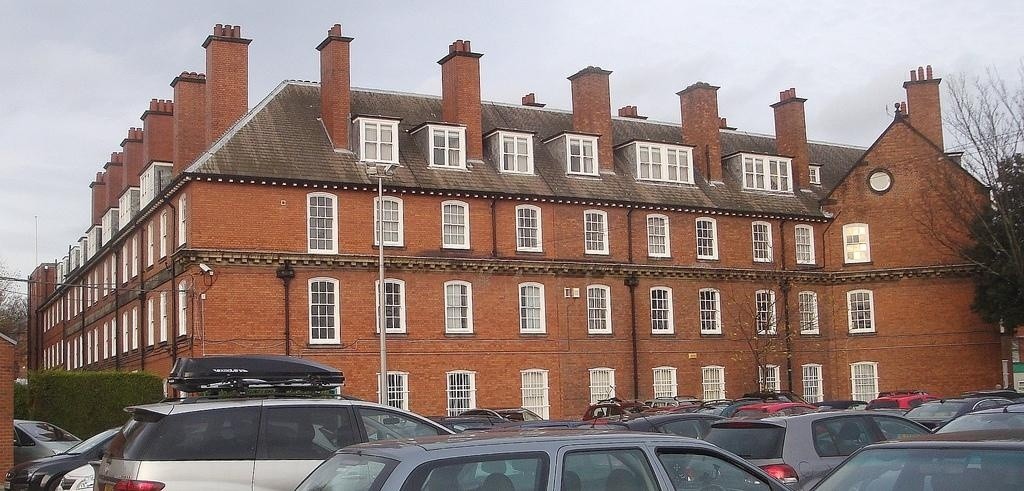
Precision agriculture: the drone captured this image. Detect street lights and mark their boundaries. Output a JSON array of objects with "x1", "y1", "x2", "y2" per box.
[{"x1": 360, "y1": 158, "x2": 406, "y2": 408}]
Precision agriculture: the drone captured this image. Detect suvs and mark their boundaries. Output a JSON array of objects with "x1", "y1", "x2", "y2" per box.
[
  {"x1": 685, "y1": 409, "x2": 934, "y2": 491},
  {"x1": 94, "y1": 353, "x2": 522, "y2": 491}
]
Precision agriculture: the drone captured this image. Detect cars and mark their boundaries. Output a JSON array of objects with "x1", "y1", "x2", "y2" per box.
[
  {"x1": 807, "y1": 428, "x2": 1024, "y2": 491},
  {"x1": 55, "y1": 459, "x2": 102, "y2": 491},
  {"x1": 295, "y1": 427, "x2": 793, "y2": 490},
  {"x1": 6, "y1": 415, "x2": 124, "y2": 491},
  {"x1": 267, "y1": 390, "x2": 1024, "y2": 453},
  {"x1": 932, "y1": 402, "x2": 1024, "y2": 434},
  {"x1": 4, "y1": 425, "x2": 124, "y2": 491}
]
